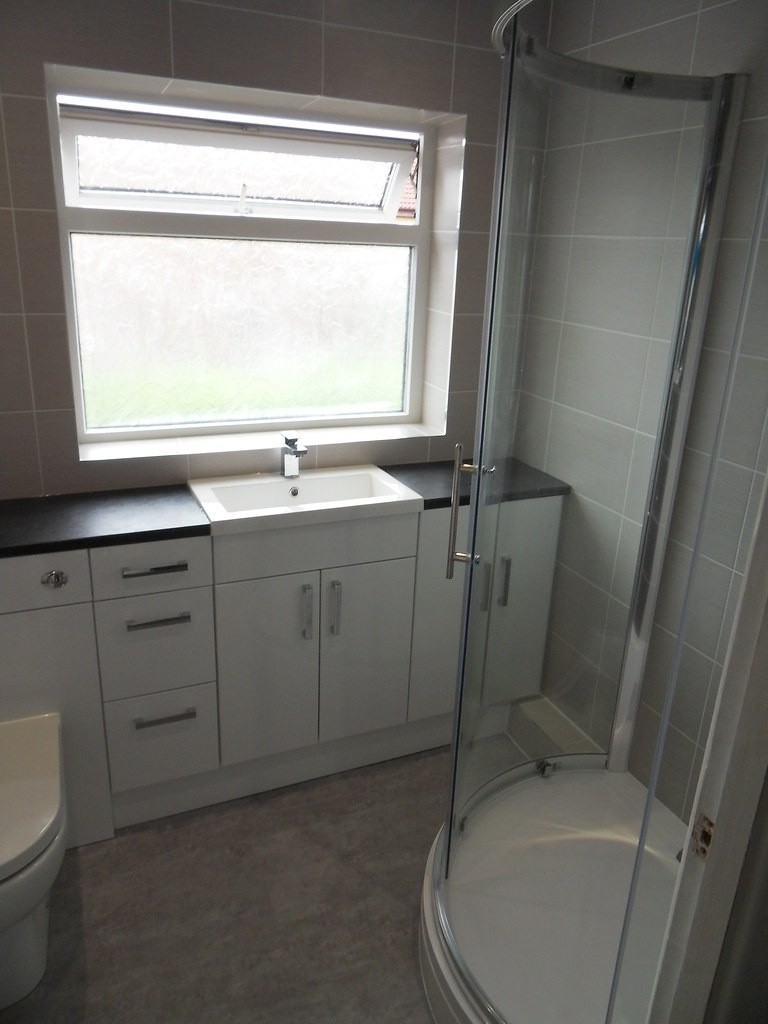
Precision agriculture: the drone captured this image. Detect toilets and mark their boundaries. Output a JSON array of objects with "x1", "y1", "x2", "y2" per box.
[{"x1": 0, "y1": 710, "x2": 67, "y2": 1012}]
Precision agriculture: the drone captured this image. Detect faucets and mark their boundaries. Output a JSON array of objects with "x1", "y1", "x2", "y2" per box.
[{"x1": 279, "y1": 430, "x2": 308, "y2": 477}]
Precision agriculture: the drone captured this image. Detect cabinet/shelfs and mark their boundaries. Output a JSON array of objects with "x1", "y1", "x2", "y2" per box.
[{"x1": 0, "y1": 493, "x2": 565, "y2": 849}]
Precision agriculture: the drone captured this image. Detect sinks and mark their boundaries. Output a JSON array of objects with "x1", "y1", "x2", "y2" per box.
[{"x1": 186, "y1": 462, "x2": 423, "y2": 522}]
